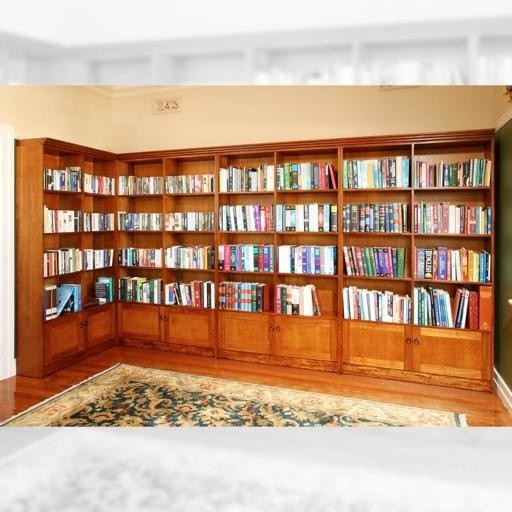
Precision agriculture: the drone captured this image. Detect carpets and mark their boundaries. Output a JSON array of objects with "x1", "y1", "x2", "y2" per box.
[{"x1": 0, "y1": 363, "x2": 468, "y2": 426}]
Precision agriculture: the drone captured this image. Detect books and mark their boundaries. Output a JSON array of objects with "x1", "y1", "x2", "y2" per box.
[
  {"x1": 118, "y1": 174, "x2": 215, "y2": 309},
  {"x1": 342, "y1": 155, "x2": 413, "y2": 324},
  {"x1": 45, "y1": 164, "x2": 115, "y2": 323},
  {"x1": 414, "y1": 158, "x2": 490, "y2": 333},
  {"x1": 218, "y1": 164, "x2": 338, "y2": 318}
]
